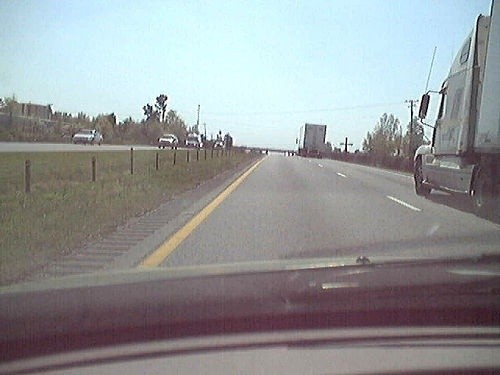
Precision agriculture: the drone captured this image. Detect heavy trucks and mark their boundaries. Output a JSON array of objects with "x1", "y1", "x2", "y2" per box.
[
  {"x1": 412, "y1": 1, "x2": 500, "y2": 222},
  {"x1": 296, "y1": 123, "x2": 327, "y2": 158}
]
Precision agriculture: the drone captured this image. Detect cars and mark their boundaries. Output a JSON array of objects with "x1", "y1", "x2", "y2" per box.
[
  {"x1": 159, "y1": 134, "x2": 179, "y2": 149},
  {"x1": 71, "y1": 129, "x2": 103, "y2": 145}
]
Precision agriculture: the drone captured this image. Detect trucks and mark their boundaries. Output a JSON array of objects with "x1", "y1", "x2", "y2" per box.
[{"x1": 185, "y1": 133, "x2": 202, "y2": 148}]
